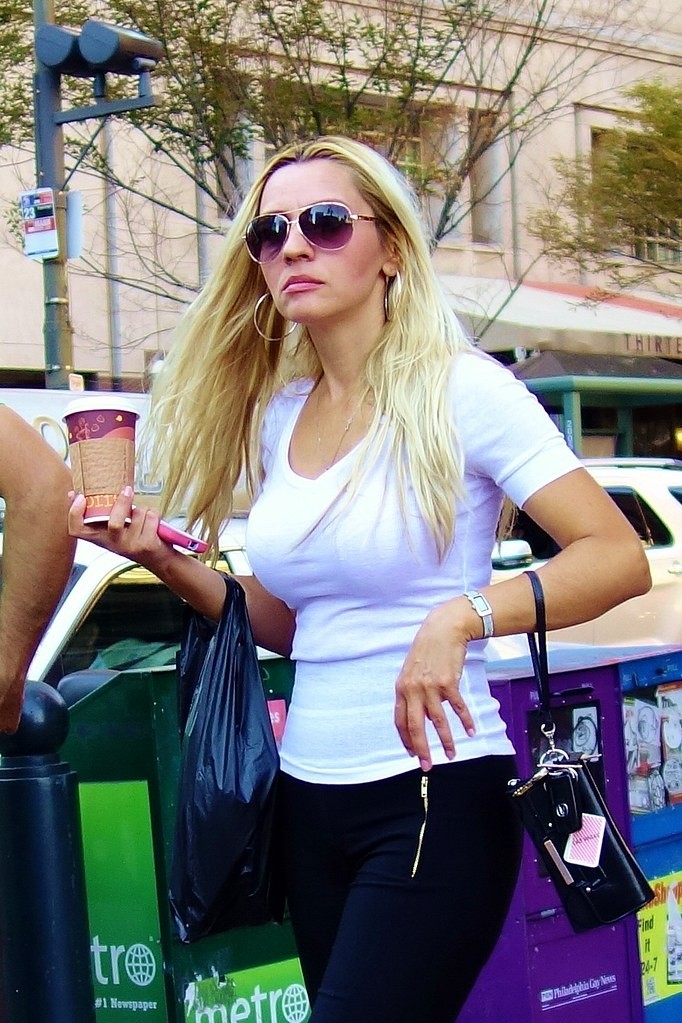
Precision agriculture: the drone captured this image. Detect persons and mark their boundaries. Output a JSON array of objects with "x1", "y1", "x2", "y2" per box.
[
  {"x1": 68, "y1": 137, "x2": 653, "y2": 1023},
  {"x1": 44, "y1": 603, "x2": 105, "y2": 689},
  {"x1": 0, "y1": 405, "x2": 79, "y2": 738}
]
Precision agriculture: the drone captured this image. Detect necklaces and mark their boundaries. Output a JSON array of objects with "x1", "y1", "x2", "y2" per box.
[{"x1": 316, "y1": 378, "x2": 366, "y2": 472}]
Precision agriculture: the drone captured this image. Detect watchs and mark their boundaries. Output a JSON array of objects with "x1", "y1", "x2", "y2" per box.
[{"x1": 464, "y1": 592, "x2": 493, "y2": 638}]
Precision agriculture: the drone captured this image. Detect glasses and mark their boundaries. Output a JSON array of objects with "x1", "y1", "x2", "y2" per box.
[{"x1": 242, "y1": 201, "x2": 382, "y2": 264}]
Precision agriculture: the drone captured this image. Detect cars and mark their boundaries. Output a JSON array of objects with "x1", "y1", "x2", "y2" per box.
[
  {"x1": 22, "y1": 516, "x2": 293, "y2": 711},
  {"x1": 483, "y1": 452, "x2": 682, "y2": 650}
]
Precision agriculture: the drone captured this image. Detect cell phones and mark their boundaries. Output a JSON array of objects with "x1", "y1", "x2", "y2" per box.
[{"x1": 131, "y1": 504, "x2": 208, "y2": 555}]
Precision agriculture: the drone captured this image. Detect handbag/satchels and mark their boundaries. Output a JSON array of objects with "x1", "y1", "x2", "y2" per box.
[
  {"x1": 166, "y1": 567, "x2": 288, "y2": 942},
  {"x1": 508, "y1": 572, "x2": 656, "y2": 932}
]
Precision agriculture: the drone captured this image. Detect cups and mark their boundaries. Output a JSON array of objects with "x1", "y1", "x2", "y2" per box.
[{"x1": 62, "y1": 397, "x2": 142, "y2": 525}]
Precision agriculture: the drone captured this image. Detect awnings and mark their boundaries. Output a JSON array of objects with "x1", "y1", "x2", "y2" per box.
[{"x1": 431, "y1": 272, "x2": 682, "y2": 354}]
